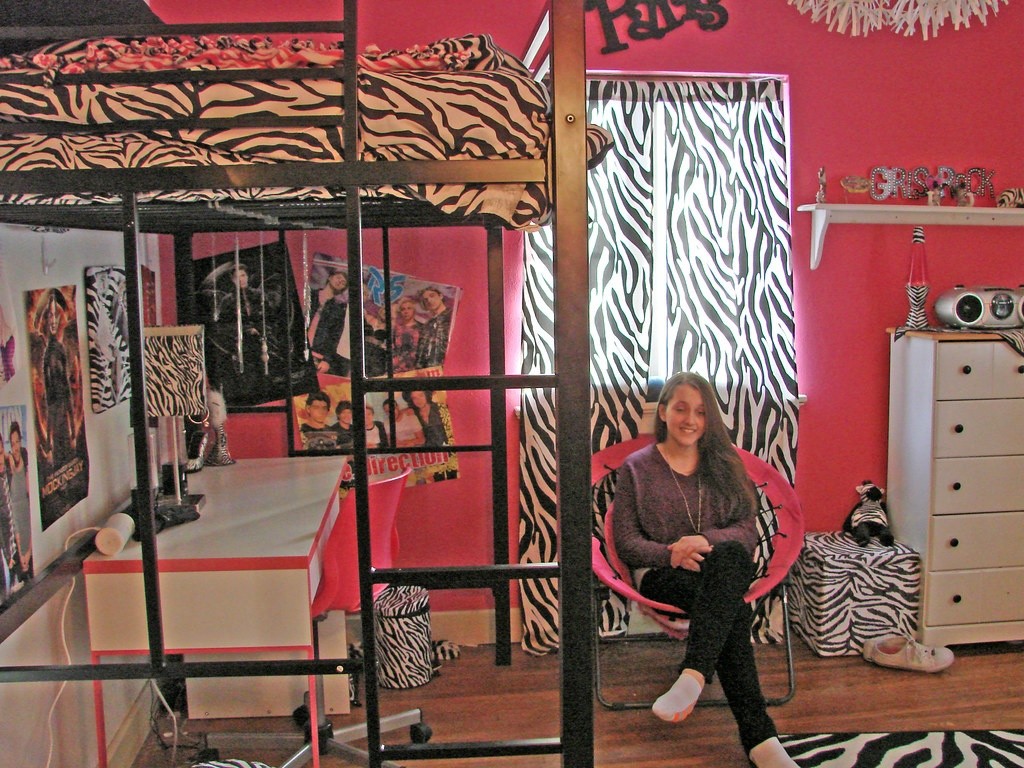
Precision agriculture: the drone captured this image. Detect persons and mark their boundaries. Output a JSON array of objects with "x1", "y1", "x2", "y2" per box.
[
  {"x1": 224, "y1": 265, "x2": 279, "y2": 362},
  {"x1": 301, "y1": 390, "x2": 447, "y2": 488},
  {"x1": 0, "y1": 423, "x2": 33, "y2": 603},
  {"x1": 308, "y1": 270, "x2": 453, "y2": 378},
  {"x1": 41, "y1": 297, "x2": 75, "y2": 491},
  {"x1": 616, "y1": 372, "x2": 800, "y2": 768}
]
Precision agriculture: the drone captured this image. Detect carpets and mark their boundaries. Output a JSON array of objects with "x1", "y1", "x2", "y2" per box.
[{"x1": 776, "y1": 729, "x2": 1024, "y2": 768}]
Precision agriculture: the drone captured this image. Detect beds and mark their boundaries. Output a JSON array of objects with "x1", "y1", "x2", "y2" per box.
[{"x1": 0, "y1": 0, "x2": 593, "y2": 768}]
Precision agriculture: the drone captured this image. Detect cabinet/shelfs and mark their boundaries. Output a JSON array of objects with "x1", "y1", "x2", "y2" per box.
[{"x1": 886, "y1": 326, "x2": 1024, "y2": 648}]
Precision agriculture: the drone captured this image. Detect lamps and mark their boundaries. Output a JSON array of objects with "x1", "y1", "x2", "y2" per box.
[{"x1": 143, "y1": 324, "x2": 208, "y2": 513}]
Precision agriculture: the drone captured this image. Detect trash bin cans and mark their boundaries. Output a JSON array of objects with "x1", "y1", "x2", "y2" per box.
[{"x1": 373, "y1": 585, "x2": 433, "y2": 689}]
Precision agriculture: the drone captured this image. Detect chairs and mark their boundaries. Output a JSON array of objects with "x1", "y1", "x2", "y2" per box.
[
  {"x1": 196, "y1": 464, "x2": 432, "y2": 768},
  {"x1": 591, "y1": 434, "x2": 805, "y2": 709}
]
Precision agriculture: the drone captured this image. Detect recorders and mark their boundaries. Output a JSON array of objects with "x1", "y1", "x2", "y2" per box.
[{"x1": 935, "y1": 283, "x2": 1024, "y2": 330}]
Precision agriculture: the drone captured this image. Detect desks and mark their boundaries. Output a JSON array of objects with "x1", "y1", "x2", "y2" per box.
[{"x1": 82, "y1": 455, "x2": 349, "y2": 768}]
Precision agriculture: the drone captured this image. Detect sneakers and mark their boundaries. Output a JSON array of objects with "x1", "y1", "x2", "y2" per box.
[{"x1": 863, "y1": 632, "x2": 955, "y2": 672}]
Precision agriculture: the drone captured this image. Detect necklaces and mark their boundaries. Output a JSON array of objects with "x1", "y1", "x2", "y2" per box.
[{"x1": 666, "y1": 444, "x2": 701, "y2": 533}]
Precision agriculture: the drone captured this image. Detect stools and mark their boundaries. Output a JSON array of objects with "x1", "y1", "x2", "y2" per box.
[{"x1": 787, "y1": 530, "x2": 920, "y2": 658}]
